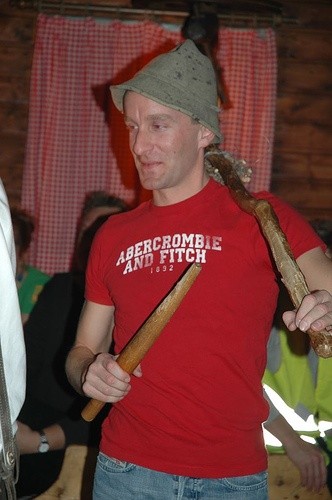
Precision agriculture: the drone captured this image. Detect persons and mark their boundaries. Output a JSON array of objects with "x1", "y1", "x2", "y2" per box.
[{"x1": 0, "y1": 36, "x2": 332, "y2": 500}]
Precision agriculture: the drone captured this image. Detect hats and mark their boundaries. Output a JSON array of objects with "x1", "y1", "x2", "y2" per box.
[{"x1": 109, "y1": 39, "x2": 223, "y2": 143}]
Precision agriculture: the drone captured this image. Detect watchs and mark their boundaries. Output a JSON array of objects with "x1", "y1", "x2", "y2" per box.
[{"x1": 36, "y1": 429, "x2": 49, "y2": 454}]
